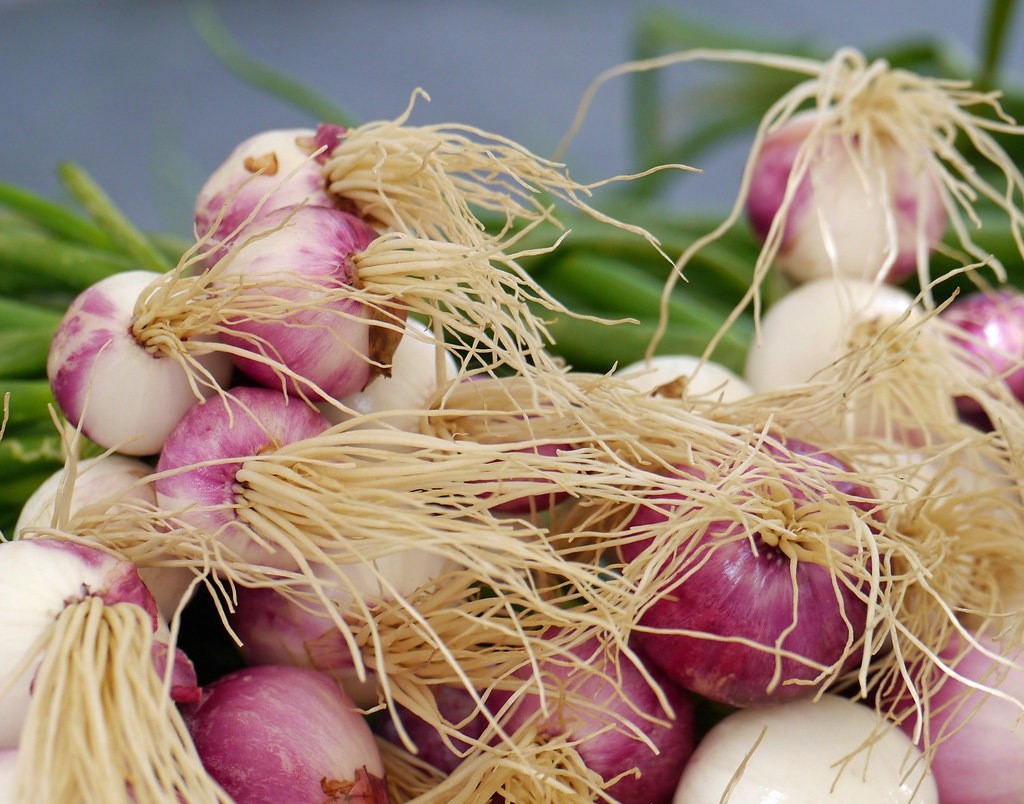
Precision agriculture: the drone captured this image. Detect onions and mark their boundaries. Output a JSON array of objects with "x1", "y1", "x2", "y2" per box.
[{"x1": 1, "y1": 45, "x2": 1024, "y2": 804}]
